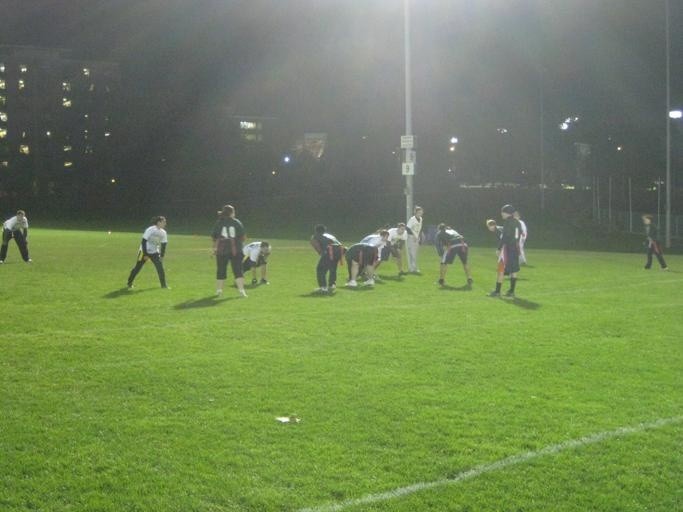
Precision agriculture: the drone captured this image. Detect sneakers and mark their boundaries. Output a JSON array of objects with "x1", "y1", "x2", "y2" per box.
[
  {"x1": 215, "y1": 278, "x2": 268, "y2": 298},
  {"x1": 468, "y1": 279, "x2": 474, "y2": 285},
  {"x1": 439, "y1": 279, "x2": 445, "y2": 286},
  {"x1": 314, "y1": 268, "x2": 420, "y2": 292},
  {"x1": 487, "y1": 291, "x2": 502, "y2": 297},
  {"x1": 505, "y1": 290, "x2": 515, "y2": 296}
]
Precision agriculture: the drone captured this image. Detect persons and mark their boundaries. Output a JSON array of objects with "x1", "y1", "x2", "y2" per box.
[
  {"x1": 241, "y1": 240, "x2": 275, "y2": 287},
  {"x1": 0, "y1": 208, "x2": 34, "y2": 265},
  {"x1": 125, "y1": 214, "x2": 172, "y2": 290},
  {"x1": 639, "y1": 210, "x2": 670, "y2": 271},
  {"x1": 309, "y1": 205, "x2": 533, "y2": 298},
  {"x1": 206, "y1": 202, "x2": 252, "y2": 300}
]
